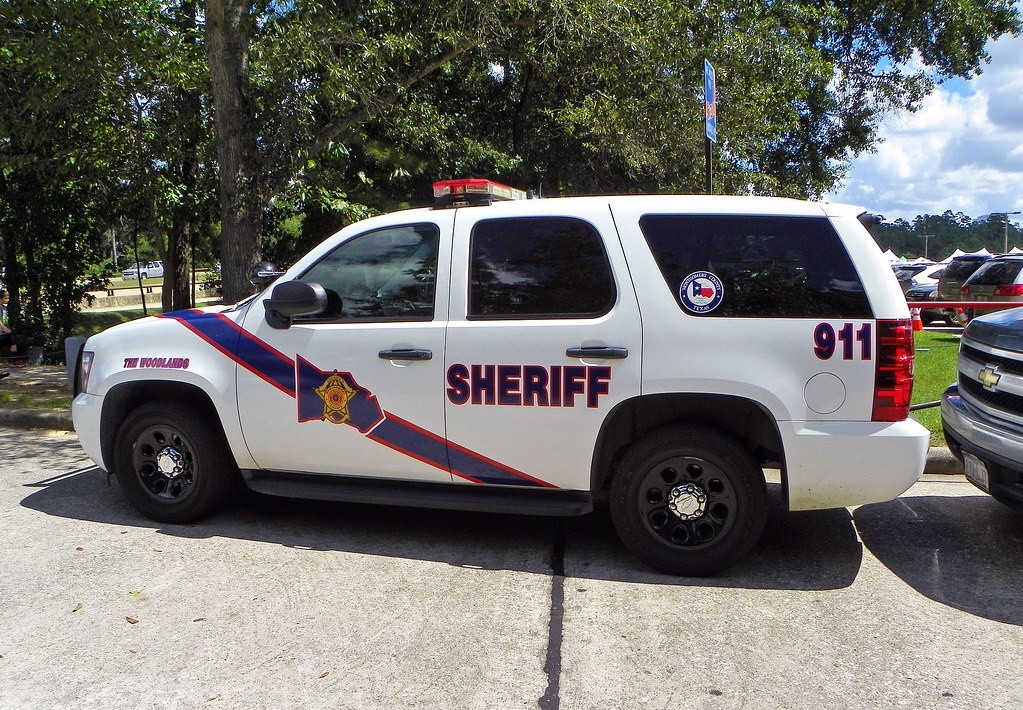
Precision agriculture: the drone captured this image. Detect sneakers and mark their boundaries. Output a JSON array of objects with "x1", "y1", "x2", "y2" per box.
[{"x1": 11, "y1": 360, "x2": 26, "y2": 368}]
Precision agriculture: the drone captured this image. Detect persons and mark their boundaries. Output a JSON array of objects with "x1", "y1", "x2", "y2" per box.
[{"x1": 0, "y1": 289, "x2": 11, "y2": 379}]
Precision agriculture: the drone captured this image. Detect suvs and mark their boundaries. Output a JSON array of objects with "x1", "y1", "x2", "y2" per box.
[
  {"x1": 940, "y1": 306, "x2": 1022, "y2": 512},
  {"x1": 960, "y1": 253, "x2": 1023, "y2": 321},
  {"x1": 122, "y1": 261, "x2": 164, "y2": 281},
  {"x1": 938, "y1": 254, "x2": 994, "y2": 313},
  {"x1": 64, "y1": 177, "x2": 932, "y2": 578}
]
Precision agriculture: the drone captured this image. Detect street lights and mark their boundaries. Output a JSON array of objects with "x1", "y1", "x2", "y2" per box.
[
  {"x1": 918, "y1": 234, "x2": 937, "y2": 259},
  {"x1": 991, "y1": 211, "x2": 1021, "y2": 255}
]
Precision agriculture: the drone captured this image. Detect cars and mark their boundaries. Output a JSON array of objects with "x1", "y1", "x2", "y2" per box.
[{"x1": 890, "y1": 261, "x2": 963, "y2": 327}]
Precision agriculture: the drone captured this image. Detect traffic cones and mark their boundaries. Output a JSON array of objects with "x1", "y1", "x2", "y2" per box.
[{"x1": 912, "y1": 308, "x2": 923, "y2": 332}]
